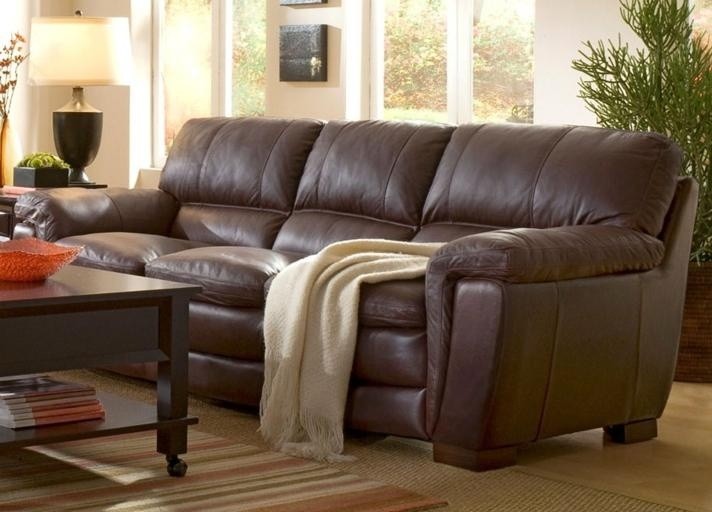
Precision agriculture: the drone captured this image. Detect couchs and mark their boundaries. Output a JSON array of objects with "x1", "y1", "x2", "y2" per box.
[{"x1": 14, "y1": 116, "x2": 699, "y2": 472}]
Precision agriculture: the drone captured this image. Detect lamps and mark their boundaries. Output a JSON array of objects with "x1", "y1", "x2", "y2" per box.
[{"x1": 27, "y1": 11, "x2": 133, "y2": 188}]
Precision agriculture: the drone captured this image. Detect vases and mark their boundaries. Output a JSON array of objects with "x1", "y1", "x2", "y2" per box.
[{"x1": 0, "y1": 116, "x2": 15, "y2": 188}]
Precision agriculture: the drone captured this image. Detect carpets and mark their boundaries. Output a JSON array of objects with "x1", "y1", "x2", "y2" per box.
[{"x1": 1, "y1": 427, "x2": 453, "y2": 512}]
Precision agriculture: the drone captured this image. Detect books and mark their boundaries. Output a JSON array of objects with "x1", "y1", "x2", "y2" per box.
[{"x1": 0, "y1": 373, "x2": 107, "y2": 431}]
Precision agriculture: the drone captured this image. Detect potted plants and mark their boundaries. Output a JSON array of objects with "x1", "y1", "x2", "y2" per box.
[{"x1": 571, "y1": 0, "x2": 712, "y2": 385}]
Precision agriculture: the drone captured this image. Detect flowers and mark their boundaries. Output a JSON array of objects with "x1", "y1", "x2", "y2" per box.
[{"x1": 0, "y1": 29, "x2": 30, "y2": 129}]
